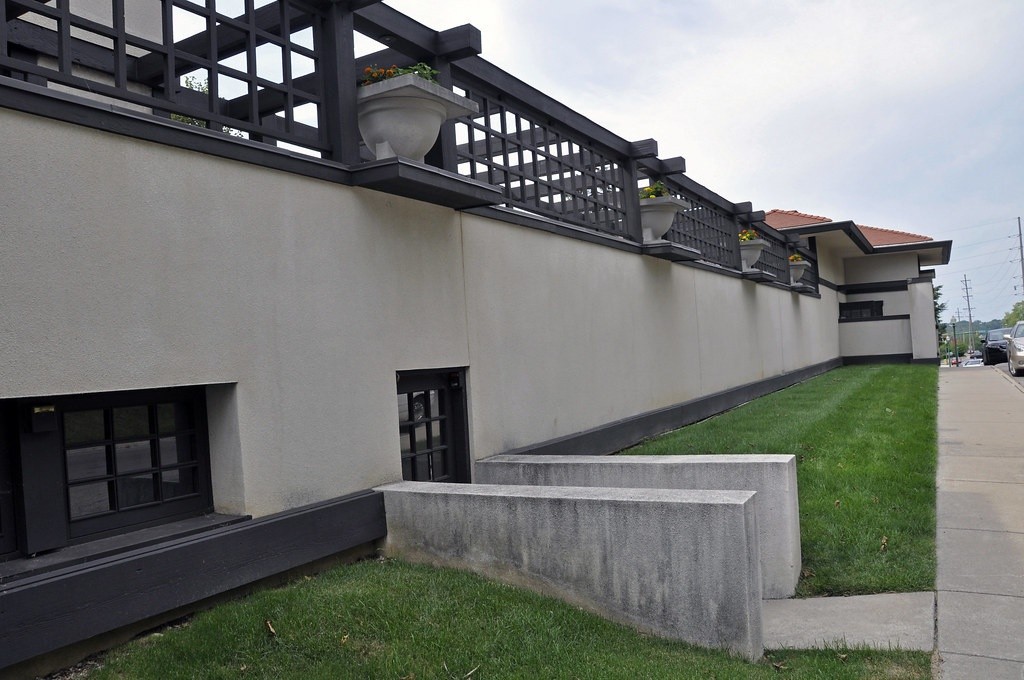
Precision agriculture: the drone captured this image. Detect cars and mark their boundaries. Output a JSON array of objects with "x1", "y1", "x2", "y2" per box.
[
  {"x1": 970, "y1": 351, "x2": 982, "y2": 359},
  {"x1": 1003, "y1": 320, "x2": 1024, "y2": 377},
  {"x1": 951, "y1": 356, "x2": 962, "y2": 365},
  {"x1": 980, "y1": 327, "x2": 1013, "y2": 366}
]
